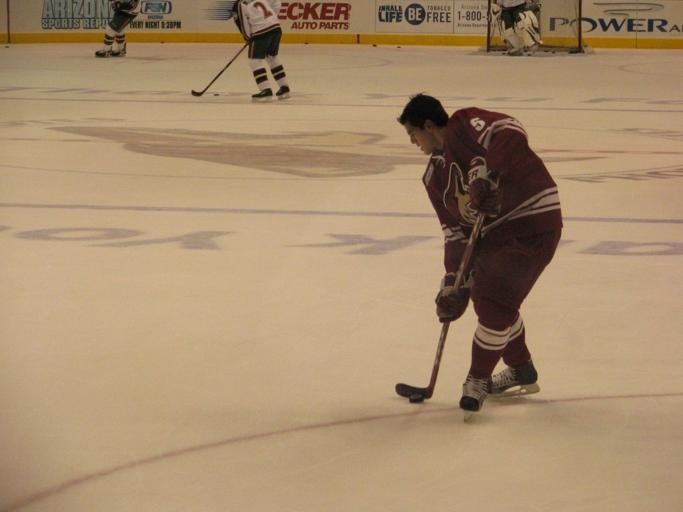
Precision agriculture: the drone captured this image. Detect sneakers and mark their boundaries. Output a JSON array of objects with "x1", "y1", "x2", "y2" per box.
[
  {"x1": 489, "y1": 359, "x2": 538, "y2": 394},
  {"x1": 460, "y1": 369, "x2": 492, "y2": 411},
  {"x1": 276, "y1": 85, "x2": 290, "y2": 96},
  {"x1": 251, "y1": 88, "x2": 273, "y2": 98}
]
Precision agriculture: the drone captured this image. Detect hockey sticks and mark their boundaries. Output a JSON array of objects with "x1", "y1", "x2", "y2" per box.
[
  {"x1": 191, "y1": 43, "x2": 248, "y2": 96},
  {"x1": 396, "y1": 210, "x2": 488, "y2": 399}
]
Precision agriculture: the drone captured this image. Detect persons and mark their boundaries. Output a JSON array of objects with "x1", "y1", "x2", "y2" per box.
[
  {"x1": 492, "y1": 0, "x2": 543, "y2": 55},
  {"x1": 396, "y1": 91, "x2": 562, "y2": 423},
  {"x1": 95, "y1": 0, "x2": 143, "y2": 57},
  {"x1": 232, "y1": 0, "x2": 292, "y2": 104}
]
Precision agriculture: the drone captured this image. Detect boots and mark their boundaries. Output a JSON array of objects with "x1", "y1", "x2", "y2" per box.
[
  {"x1": 114, "y1": 42, "x2": 127, "y2": 56},
  {"x1": 95, "y1": 45, "x2": 113, "y2": 57}
]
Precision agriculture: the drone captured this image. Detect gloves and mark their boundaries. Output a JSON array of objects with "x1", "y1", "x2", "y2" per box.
[
  {"x1": 468, "y1": 166, "x2": 505, "y2": 214},
  {"x1": 435, "y1": 272, "x2": 472, "y2": 324}
]
